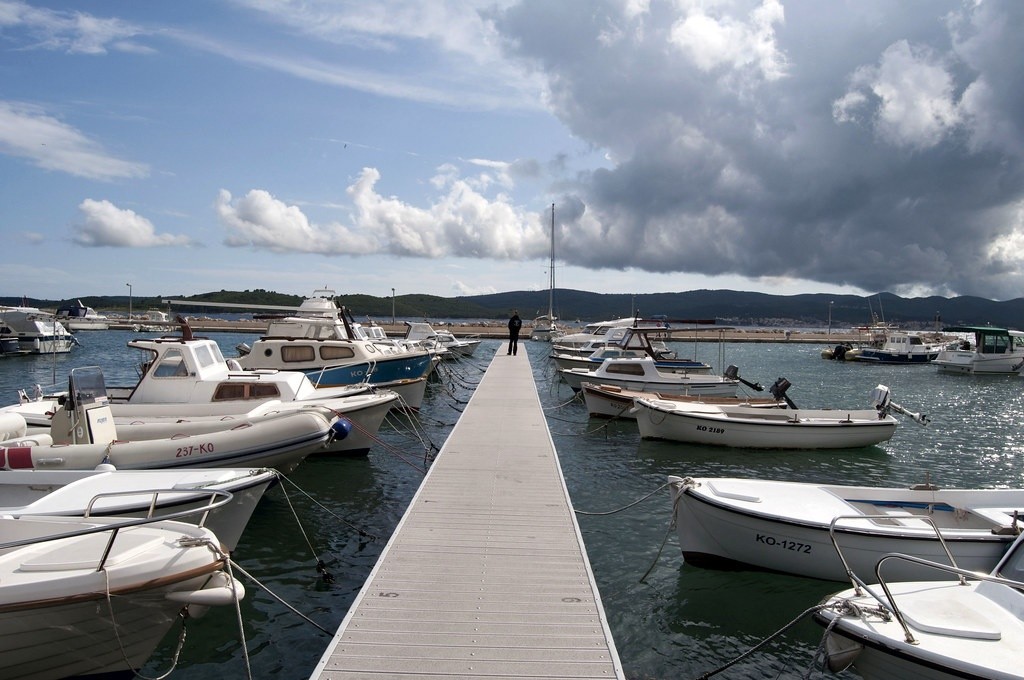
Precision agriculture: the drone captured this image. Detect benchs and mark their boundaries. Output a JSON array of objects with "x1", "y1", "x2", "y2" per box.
[
  {"x1": 874, "y1": 505, "x2": 931, "y2": 527},
  {"x1": 965, "y1": 505, "x2": 1024, "y2": 526}
]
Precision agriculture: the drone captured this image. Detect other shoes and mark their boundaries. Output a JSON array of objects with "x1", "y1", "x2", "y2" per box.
[
  {"x1": 514, "y1": 354, "x2": 516, "y2": 355},
  {"x1": 507, "y1": 353, "x2": 511, "y2": 355}
]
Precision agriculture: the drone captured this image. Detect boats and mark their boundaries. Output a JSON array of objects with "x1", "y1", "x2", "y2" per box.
[
  {"x1": 0, "y1": 514, "x2": 243, "y2": 680},
  {"x1": 0, "y1": 367, "x2": 342, "y2": 488},
  {"x1": 855, "y1": 334, "x2": 962, "y2": 362},
  {"x1": 0, "y1": 337, "x2": 398, "y2": 461},
  {"x1": 55, "y1": 305, "x2": 111, "y2": 331},
  {"x1": 545, "y1": 317, "x2": 712, "y2": 377},
  {"x1": 930, "y1": 326, "x2": 1024, "y2": 374},
  {"x1": 822, "y1": 346, "x2": 858, "y2": 359},
  {"x1": 557, "y1": 356, "x2": 741, "y2": 404},
  {"x1": 144, "y1": 299, "x2": 436, "y2": 414},
  {"x1": 137, "y1": 310, "x2": 176, "y2": 331},
  {"x1": 633, "y1": 394, "x2": 898, "y2": 447},
  {"x1": 668, "y1": 476, "x2": 1024, "y2": 582},
  {"x1": 812, "y1": 526, "x2": 1024, "y2": 680},
  {"x1": 0, "y1": 469, "x2": 277, "y2": 555},
  {"x1": 0, "y1": 306, "x2": 75, "y2": 354},
  {"x1": 579, "y1": 382, "x2": 787, "y2": 424},
  {"x1": 359, "y1": 322, "x2": 482, "y2": 372}
]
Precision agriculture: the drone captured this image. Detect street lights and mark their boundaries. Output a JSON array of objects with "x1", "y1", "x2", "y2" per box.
[
  {"x1": 126, "y1": 283, "x2": 131, "y2": 320},
  {"x1": 828, "y1": 301, "x2": 834, "y2": 334},
  {"x1": 936, "y1": 311, "x2": 940, "y2": 331},
  {"x1": 392, "y1": 288, "x2": 395, "y2": 325},
  {"x1": 631, "y1": 296, "x2": 636, "y2": 316}
]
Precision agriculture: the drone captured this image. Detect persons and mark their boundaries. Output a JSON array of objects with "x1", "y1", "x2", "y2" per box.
[{"x1": 507, "y1": 310, "x2": 522, "y2": 355}]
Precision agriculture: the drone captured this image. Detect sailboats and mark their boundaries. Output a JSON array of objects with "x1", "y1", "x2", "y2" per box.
[{"x1": 531, "y1": 203, "x2": 566, "y2": 341}]
